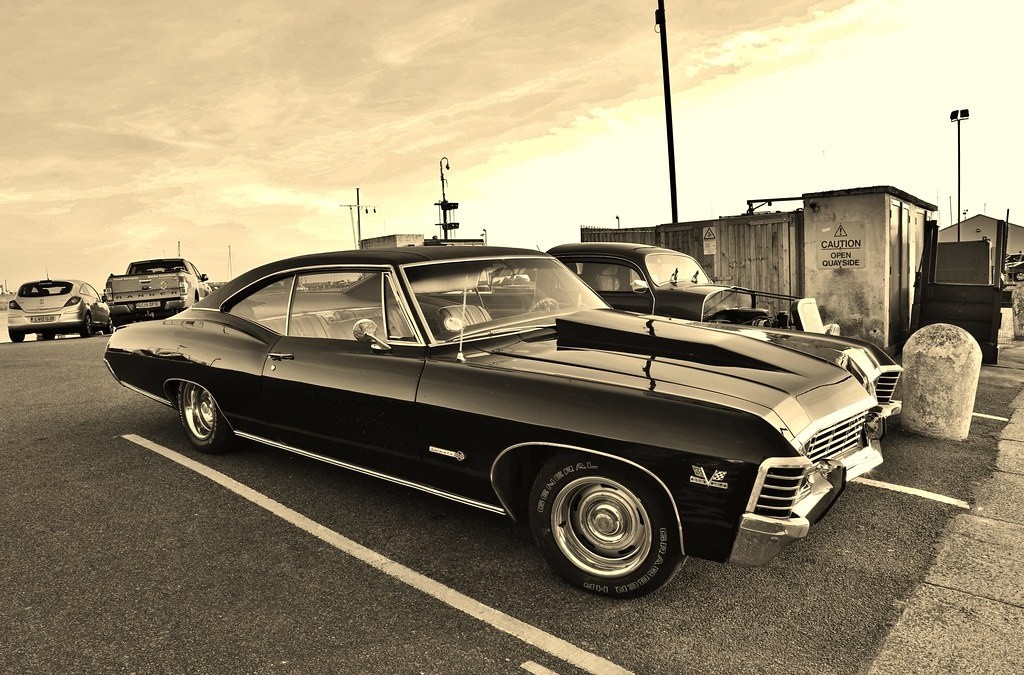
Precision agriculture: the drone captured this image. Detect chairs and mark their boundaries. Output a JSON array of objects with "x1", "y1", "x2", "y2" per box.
[
  {"x1": 439, "y1": 303, "x2": 492, "y2": 334},
  {"x1": 39, "y1": 289, "x2": 50, "y2": 296},
  {"x1": 582, "y1": 265, "x2": 621, "y2": 291}
]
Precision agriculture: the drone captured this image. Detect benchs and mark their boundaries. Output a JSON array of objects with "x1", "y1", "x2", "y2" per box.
[{"x1": 260, "y1": 307, "x2": 417, "y2": 341}]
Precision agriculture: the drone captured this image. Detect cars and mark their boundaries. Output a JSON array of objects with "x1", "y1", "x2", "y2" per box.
[
  {"x1": 414, "y1": 241, "x2": 905, "y2": 431},
  {"x1": 105, "y1": 245, "x2": 886, "y2": 601},
  {"x1": 7, "y1": 279, "x2": 113, "y2": 343},
  {"x1": 1005, "y1": 253, "x2": 1024, "y2": 281}
]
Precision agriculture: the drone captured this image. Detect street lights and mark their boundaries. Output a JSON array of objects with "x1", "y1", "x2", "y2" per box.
[
  {"x1": 439, "y1": 155, "x2": 460, "y2": 240},
  {"x1": 950, "y1": 109, "x2": 971, "y2": 242}
]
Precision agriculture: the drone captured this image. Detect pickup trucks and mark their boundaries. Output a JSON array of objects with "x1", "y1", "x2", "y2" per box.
[{"x1": 104, "y1": 258, "x2": 213, "y2": 329}]
